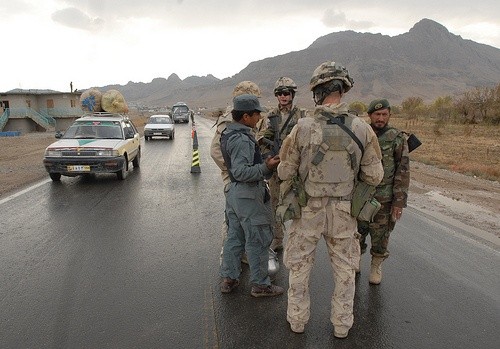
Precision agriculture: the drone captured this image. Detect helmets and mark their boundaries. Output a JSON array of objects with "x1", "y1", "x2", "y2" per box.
[
  {"x1": 232, "y1": 81, "x2": 262, "y2": 99},
  {"x1": 274, "y1": 77, "x2": 298, "y2": 95},
  {"x1": 309, "y1": 61, "x2": 354, "y2": 93}
]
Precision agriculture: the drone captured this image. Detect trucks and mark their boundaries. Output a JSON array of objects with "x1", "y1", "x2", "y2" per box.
[{"x1": 171, "y1": 103, "x2": 191, "y2": 123}]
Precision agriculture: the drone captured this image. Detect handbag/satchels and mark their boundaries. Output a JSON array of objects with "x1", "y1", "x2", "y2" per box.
[
  {"x1": 275, "y1": 176, "x2": 306, "y2": 222},
  {"x1": 350, "y1": 182, "x2": 381, "y2": 223}
]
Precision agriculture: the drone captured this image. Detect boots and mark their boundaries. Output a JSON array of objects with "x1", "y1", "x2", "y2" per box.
[{"x1": 368, "y1": 255, "x2": 386, "y2": 285}]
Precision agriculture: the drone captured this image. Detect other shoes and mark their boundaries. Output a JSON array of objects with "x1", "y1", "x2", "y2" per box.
[
  {"x1": 240, "y1": 251, "x2": 248, "y2": 263},
  {"x1": 334, "y1": 323, "x2": 349, "y2": 338},
  {"x1": 220, "y1": 278, "x2": 240, "y2": 292},
  {"x1": 267, "y1": 258, "x2": 281, "y2": 273},
  {"x1": 251, "y1": 282, "x2": 284, "y2": 296},
  {"x1": 290, "y1": 320, "x2": 305, "y2": 333}
]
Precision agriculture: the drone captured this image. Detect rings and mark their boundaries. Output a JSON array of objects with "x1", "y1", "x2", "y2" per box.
[{"x1": 399, "y1": 212, "x2": 401, "y2": 213}]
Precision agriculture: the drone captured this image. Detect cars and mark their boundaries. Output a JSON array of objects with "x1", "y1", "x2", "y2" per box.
[
  {"x1": 42, "y1": 112, "x2": 142, "y2": 181},
  {"x1": 144, "y1": 115, "x2": 176, "y2": 140}
]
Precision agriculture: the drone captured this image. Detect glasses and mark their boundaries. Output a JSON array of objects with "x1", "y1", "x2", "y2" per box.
[{"x1": 277, "y1": 91, "x2": 292, "y2": 97}]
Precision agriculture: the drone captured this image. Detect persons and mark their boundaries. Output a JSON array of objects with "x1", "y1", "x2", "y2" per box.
[
  {"x1": 277, "y1": 60, "x2": 385, "y2": 338},
  {"x1": 354, "y1": 97, "x2": 410, "y2": 285},
  {"x1": 210, "y1": 77, "x2": 305, "y2": 298}
]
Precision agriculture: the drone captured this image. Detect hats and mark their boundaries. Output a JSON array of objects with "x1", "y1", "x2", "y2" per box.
[
  {"x1": 234, "y1": 94, "x2": 266, "y2": 112},
  {"x1": 367, "y1": 98, "x2": 391, "y2": 114}
]
